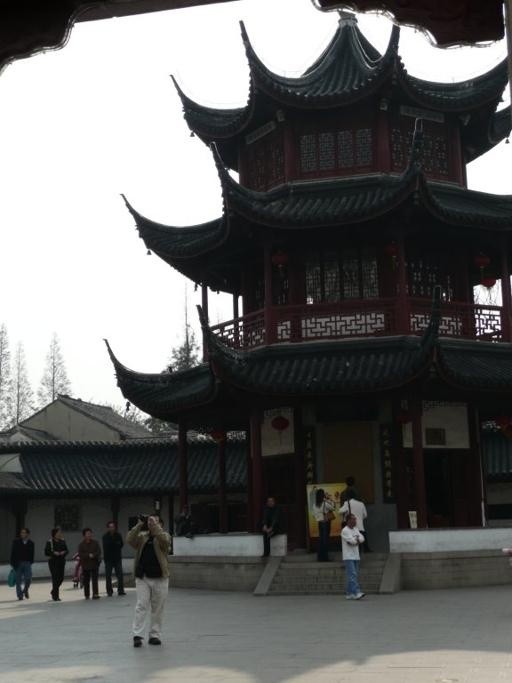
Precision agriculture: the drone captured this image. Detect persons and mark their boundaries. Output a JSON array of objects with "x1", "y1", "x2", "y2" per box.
[
  {"x1": 127, "y1": 514, "x2": 172, "y2": 648},
  {"x1": 79, "y1": 528, "x2": 101, "y2": 600},
  {"x1": 9, "y1": 527, "x2": 35, "y2": 600},
  {"x1": 175, "y1": 505, "x2": 195, "y2": 539},
  {"x1": 103, "y1": 521, "x2": 126, "y2": 596},
  {"x1": 45, "y1": 528, "x2": 70, "y2": 601},
  {"x1": 260, "y1": 493, "x2": 285, "y2": 558},
  {"x1": 313, "y1": 476, "x2": 374, "y2": 600}
]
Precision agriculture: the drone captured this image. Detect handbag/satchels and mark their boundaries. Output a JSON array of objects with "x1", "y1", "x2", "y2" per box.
[
  {"x1": 8, "y1": 571, "x2": 16, "y2": 586},
  {"x1": 324, "y1": 510, "x2": 335, "y2": 521},
  {"x1": 341, "y1": 521, "x2": 347, "y2": 528}
]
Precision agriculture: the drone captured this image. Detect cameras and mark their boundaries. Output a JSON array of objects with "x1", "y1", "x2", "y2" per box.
[{"x1": 137, "y1": 513, "x2": 149, "y2": 522}]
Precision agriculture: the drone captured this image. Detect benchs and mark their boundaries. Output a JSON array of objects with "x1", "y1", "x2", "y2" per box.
[
  {"x1": 388, "y1": 527, "x2": 512, "y2": 553},
  {"x1": 172, "y1": 533, "x2": 289, "y2": 557}
]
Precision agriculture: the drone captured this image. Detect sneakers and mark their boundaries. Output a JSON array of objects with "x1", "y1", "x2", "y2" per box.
[
  {"x1": 346, "y1": 592, "x2": 365, "y2": 600},
  {"x1": 149, "y1": 638, "x2": 161, "y2": 644},
  {"x1": 134, "y1": 637, "x2": 141, "y2": 646}
]
